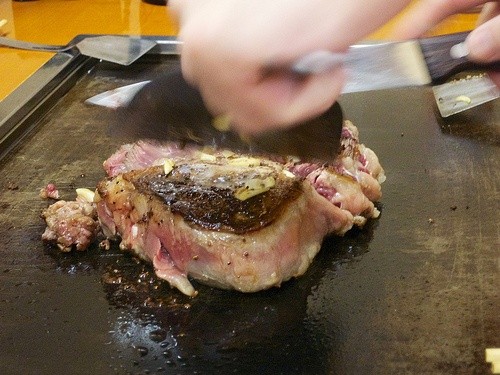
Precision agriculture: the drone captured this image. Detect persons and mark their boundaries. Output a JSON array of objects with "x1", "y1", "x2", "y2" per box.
[{"x1": 166, "y1": 1, "x2": 500, "y2": 137}]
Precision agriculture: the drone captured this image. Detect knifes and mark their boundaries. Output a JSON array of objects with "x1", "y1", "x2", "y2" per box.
[{"x1": 83, "y1": 28, "x2": 500, "y2": 112}]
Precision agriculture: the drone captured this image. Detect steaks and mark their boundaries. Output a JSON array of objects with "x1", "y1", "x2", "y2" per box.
[{"x1": 39, "y1": 119, "x2": 387, "y2": 298}]
